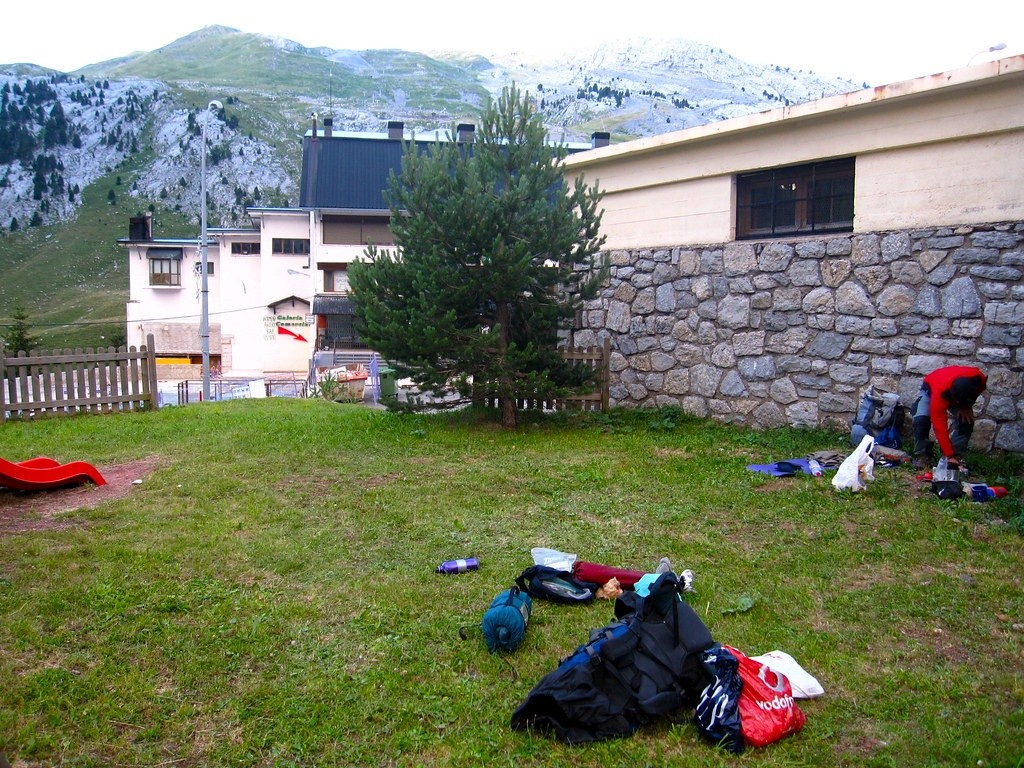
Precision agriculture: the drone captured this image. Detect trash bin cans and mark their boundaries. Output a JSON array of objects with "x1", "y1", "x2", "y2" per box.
[{"x1": 378, "y1": 369, "x2": 398, "y2": 398}]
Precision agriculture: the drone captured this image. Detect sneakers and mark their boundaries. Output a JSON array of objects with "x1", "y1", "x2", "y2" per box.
[{"x1": 680, "y1": 569, "x2": 697, "y2": 595}]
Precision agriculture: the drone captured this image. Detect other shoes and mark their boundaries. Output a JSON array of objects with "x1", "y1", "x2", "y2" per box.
[
  {"x1": 914, "y1": 456, "x2": 931, "y2": 469},
  {"x1": 955, "y1": 455, "x2": 969, "y2": 468}
]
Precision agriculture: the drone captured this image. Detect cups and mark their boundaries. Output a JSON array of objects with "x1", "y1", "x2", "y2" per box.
[{"x1": 971, "y1": 486, "x2": 988, "y2": 502}]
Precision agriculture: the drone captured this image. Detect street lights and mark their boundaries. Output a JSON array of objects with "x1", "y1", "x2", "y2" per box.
[{"x1": 198, "y1": 99, "x2": 224, "y2": 400}]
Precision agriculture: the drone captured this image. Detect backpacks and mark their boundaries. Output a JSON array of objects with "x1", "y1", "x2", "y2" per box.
[
  {"x1": 586, "y1": 573, "x2": 718, "y2": 728},
  {"x1": 850, "y1": 386, "x2": 905, "y2": 451},
  {"x1": 510, "y1": 611, "x2": 651, "y2": 743}
]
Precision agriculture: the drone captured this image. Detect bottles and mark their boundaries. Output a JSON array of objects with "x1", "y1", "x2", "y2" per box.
[
  {"x1": 434, "y1": 558, "x2": 479, "y2": 573},
  {"x1": 808, "y1": 465, "x2": 822, "y2": 477},
  {"x1": 988, "y1": 486, "x2": 1008, "y2": 498}
]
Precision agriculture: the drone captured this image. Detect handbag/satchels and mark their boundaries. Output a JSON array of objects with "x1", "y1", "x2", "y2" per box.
[
  {"x1": 510, "y1": 564, "x2": 597, "y2": 605},
  {"x1": 691, "y1": 642, "x2": 824, "y2": 754},
  {"x1": 831, "y1": 433, "x2": 874, "y2": 493},
  {"x1": 863, "y1": 424, "x2": 903, "y2": 467}
]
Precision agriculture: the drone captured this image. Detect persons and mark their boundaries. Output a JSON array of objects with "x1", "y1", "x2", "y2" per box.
[{"x1": 908, "y1": 365, "x2": 987, "y2": 467}]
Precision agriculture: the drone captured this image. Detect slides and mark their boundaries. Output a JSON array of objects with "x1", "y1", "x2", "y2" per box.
[{"x1": 0, "y1": 455, "x2": 113, "y2": 492}]
